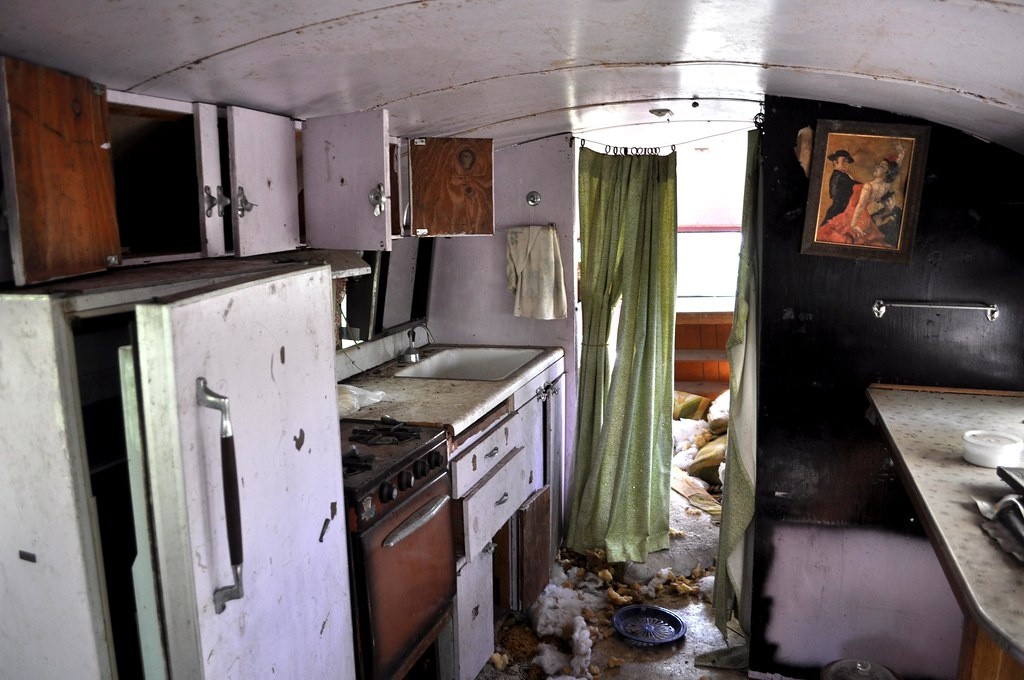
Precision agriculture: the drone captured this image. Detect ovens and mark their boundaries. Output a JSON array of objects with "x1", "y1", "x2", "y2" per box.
[{"x1": 345, "y1": 473, "x2": 461, "y2": 680}]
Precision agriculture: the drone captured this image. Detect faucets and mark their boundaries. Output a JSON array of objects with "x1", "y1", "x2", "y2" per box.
[{"x1": 403, "y1": 324, "x2": 436, "y2": 363}]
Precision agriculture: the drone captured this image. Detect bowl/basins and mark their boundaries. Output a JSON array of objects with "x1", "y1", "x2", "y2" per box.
[{"x1": 962, "y1": 430, "x2": 1023, "y2": 469}]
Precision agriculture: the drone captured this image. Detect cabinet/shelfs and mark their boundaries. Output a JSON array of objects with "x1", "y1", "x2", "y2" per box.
[
  {"x1": 0, "y1": 50, "x2": 498, "y2": 287},
  {"x1": 437, "y1": 374, "x2": 566, "y2": 680}
]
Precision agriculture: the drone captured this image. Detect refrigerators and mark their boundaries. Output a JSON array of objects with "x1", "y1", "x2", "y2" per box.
[{"x1": 0, "y1": 258, "x2": 355, "y2": 680}]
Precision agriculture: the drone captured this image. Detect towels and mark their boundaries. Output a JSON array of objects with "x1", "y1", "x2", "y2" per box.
[{"x1": 505, "y1": 224, "x2": 568, "y2": 322}]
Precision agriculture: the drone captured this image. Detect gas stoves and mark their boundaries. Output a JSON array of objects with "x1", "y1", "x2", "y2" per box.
[{"x1": 338, "y1": 416, "x2": 449, "y2": 526}]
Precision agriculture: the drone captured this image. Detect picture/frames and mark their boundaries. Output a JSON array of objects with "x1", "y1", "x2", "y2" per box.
[{"x1": 799, "y1": 117, "x2": 928, "y2": 266}]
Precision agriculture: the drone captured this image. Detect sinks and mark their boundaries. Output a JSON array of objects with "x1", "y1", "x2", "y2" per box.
[{"x1": 391, "y1": 347, "x2": 547, "y2": 381}]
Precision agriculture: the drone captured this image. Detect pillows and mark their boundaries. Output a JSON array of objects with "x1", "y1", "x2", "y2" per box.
[{"x1": 674, "y1": 390, "x2": 710, "y2": 420}]
